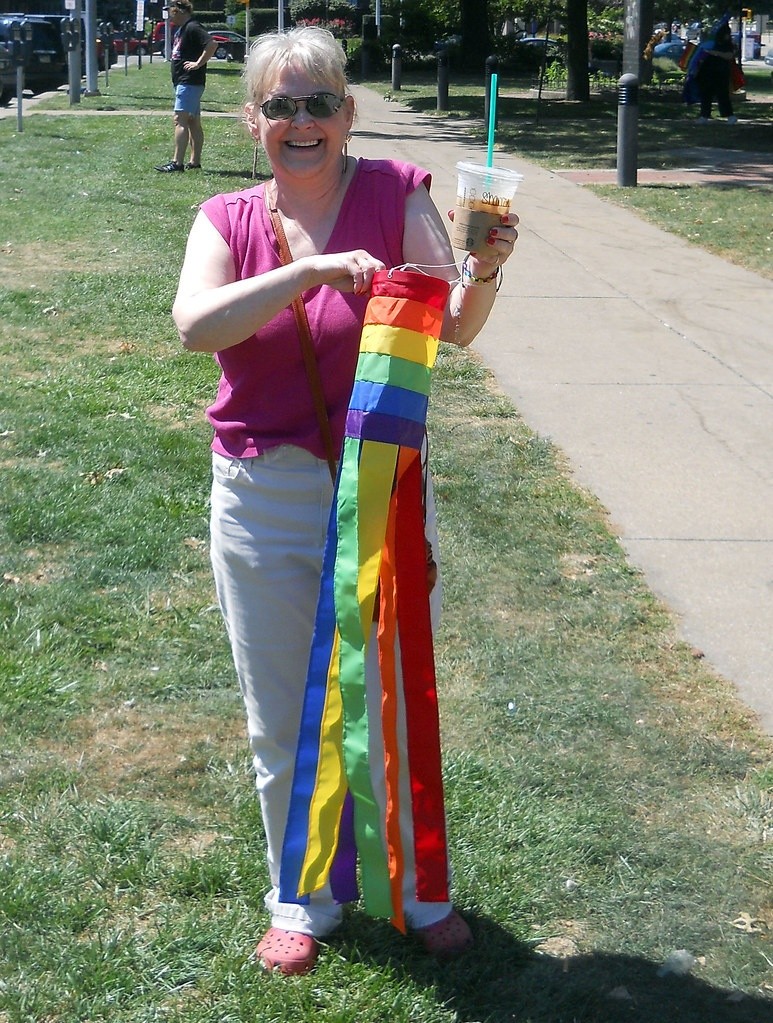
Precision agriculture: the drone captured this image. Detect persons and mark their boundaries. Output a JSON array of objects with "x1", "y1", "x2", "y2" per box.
[
  {"x1": 171, "y1": 23, "x2": 520, "y2": 972},
  {"x1": 154, "y1": 0, "x2": 218, "y2": 173},
  {"x1": 695, "y1": 23, "x2": 737, "y2": 126}
]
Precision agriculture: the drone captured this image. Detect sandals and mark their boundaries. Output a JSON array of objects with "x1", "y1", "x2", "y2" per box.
[
  {"x1": 417, "y1": 907, "x2": 473, "y2": 958},
  {"x1": 256, "y1": 927, "x2": 321, "y2": 977}
]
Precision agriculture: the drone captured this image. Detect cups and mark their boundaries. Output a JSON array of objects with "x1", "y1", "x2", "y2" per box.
[{"x1": 450, "y1": 161, "x2": 524, "y2": 252}]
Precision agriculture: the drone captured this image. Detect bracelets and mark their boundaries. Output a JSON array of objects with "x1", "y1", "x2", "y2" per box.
[{"x1": 461, "y1": 254, "x2": 503, "y2": 292}]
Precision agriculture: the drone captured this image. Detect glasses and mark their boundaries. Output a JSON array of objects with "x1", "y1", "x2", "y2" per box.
[{"x1": 255, "y1": 91, "x2": 343, "y2": 121}]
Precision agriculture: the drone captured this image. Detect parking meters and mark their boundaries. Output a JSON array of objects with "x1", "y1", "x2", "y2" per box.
[
  {"x1": 99, "y1": 21, "x2": 113, "y2": 89},
  {"x1": 7, "y1": 17, "x2": 34, "y2": 132},
  {"x1": 60, "y1": 16, "x2": 81, "y2": 111}
]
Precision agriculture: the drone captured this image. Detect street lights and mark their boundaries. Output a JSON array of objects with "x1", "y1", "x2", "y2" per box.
[{"x1": 119, "y1": 20, "x2": 134, "y2": 76}]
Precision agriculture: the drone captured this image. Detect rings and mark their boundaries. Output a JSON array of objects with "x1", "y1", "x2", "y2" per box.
[{"x1": 509, "y1": 229, "x2": 518, "y2": 244}]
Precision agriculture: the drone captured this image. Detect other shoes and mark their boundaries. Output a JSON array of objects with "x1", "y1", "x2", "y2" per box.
[
  {"x1": 725, "y1": 115, "x2": 737, "y2": 124},
  {"x1": 694, "y1": 116, "x2": 708, "y2": 124},
  {"x1": 154, "y1": 161, "x2": 184, "y2": 173},
  {"x1": 185, "y1": 162, "x2": 201, "y2": 170}
]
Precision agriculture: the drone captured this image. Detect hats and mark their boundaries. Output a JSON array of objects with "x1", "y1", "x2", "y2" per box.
[{"x1": 162, "y1": 1, "x2": 190, "y2": 11}]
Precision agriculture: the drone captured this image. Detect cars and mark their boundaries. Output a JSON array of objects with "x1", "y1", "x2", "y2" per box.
[
  {"x1": 588, "y1": 42, "x2": 625, "y2": 75},
  {"x1": 764, "y1": 47, "x2": 773, "y2": 66},
  {"x1": 651, "y1": 42, "x2": 688, "y2": 64},
  {"x1": 0, "y1": 10, "x2": 252, "y2": 105},
  {"x1": 515, "y1": 37, "x2": 563, "y2": 67}
]
